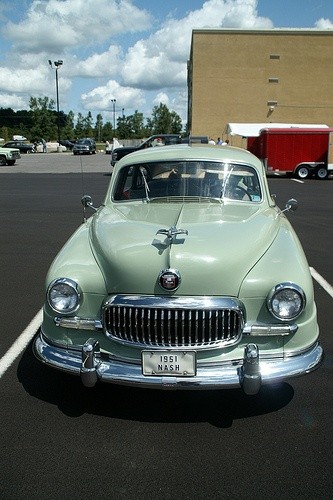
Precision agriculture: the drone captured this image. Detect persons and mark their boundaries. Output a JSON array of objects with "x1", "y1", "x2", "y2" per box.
[
  {"x1": 42, "y1": 139, "x2": 48, "y2": 153},
  {"x1": 155, "y1": 138, "x2": 164, "y2": 146},
  {"x1": 221, "y1": 139, "x2": 229, "y2": 145},
  {"x1": 209, "y1": 138, "x2": 215, "y2": 145},
  {"x1": 216, "y1": 137, "x2": 222, "y2": 145}
]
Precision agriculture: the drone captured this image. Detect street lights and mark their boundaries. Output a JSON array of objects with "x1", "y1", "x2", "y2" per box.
[
  {"x1": 48, "y1": 60, "x2": 64, "y2": 153},
  {"x1": 111, "y1": 99, "x2": 116, "y2": 138}
]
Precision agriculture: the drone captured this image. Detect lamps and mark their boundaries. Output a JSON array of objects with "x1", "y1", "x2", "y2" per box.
[{"x1": 270, "y1": 105, "x2": 274, "y2": 112}]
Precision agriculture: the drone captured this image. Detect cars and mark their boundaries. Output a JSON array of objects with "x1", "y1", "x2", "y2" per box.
[
  {"x1": 31, "y1": 142, "x2": 67, "y2": 153},
  {"x1": 56, "y1": 139, "x2": 75, "y2": 150},
  {"x1": 32, "y1": 143, "x2": 324, "y2": 396},
  {"x1": 106, "y1": 140, "x2": 124, "y2": 155}
]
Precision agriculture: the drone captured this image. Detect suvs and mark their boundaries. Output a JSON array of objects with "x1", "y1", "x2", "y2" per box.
[
  {"x1": 72, "y1": 138, "x2": 97, "y2": 155},
  {"x1": 0, "y1": 146, "x2": 21, "y2": 166}
]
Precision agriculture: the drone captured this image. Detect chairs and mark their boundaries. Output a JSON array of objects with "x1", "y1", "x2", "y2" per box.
[
  {"x1": 148, "y1": 169, "x2": 197, "y2": 198},
  {"x1": 204, "y1": 173, "x2": 246, "y2": 200}
]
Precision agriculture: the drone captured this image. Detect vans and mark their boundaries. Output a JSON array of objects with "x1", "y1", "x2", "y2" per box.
[{"x1": 110, "y1": 134, "x2": 208, "y2": 176}]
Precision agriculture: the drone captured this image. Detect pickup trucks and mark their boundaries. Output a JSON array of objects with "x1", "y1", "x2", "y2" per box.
[{"x1": 2, "y1": 140, "x2": 35, "y2": 154}]
